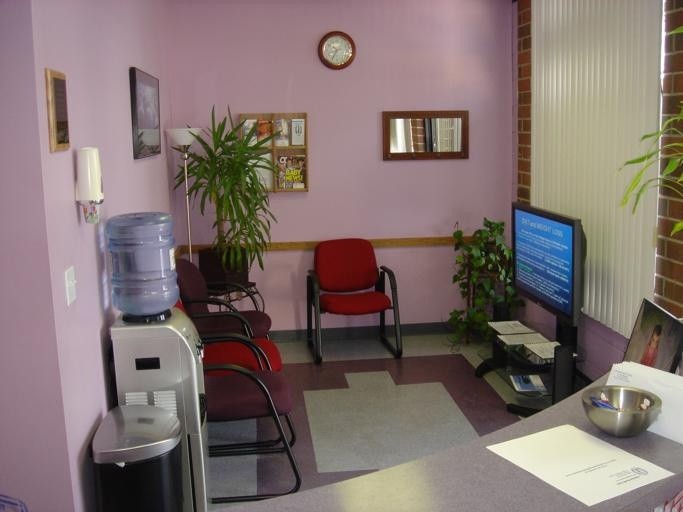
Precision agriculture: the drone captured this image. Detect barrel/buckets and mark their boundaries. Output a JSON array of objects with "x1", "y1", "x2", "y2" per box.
[{"x1": 104, "y1": 212, "x2": 180, "y2": 316}]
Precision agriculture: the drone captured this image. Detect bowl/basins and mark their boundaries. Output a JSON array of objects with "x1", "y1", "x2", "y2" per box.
[{"x1": 581, "y1": 385, "x2": 661, "y2": 438}]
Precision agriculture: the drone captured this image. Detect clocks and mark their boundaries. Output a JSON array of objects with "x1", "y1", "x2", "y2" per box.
[{"x1": 318, "y1": 31, "x2": 356, "y2": 70}]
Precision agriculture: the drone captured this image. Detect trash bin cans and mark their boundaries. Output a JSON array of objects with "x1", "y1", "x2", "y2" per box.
[{"x1": 91, "y1": 404, "x2": 182, "y2": 512}]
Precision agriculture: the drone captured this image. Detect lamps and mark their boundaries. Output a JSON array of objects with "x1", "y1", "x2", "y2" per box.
[{"x1": 166, "y1": 128, "x2": 202, "y2": 261}]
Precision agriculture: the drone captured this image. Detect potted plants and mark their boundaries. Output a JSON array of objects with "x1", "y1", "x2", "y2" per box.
[
  {"x1": 445, "y1": 216, "x2": 525, "y2": 344},
  {"x1": 173, "y1": 104, "x2": 284, "y2": 288}
]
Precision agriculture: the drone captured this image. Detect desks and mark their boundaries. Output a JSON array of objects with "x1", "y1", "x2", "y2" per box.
[{"x1": 238, "y1": 361, "x2": 683, "y2": 512}]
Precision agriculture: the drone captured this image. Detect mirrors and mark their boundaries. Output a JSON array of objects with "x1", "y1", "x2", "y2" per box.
[{"x1": 382, "y1": 110, "x2": 469, "y2": 160}]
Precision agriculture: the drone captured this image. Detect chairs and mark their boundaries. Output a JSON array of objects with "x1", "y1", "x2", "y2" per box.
[
  {"x1": 306, "y1": 239, "x2": 402, "y2": 363},
  {"x1": 175, "y1": 259, "x2": 302, "y2": 504}
]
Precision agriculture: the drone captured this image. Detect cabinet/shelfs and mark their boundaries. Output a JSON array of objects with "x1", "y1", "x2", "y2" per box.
[
  {"x1": 239, "y1": 112, "x2": 309, "y2": 193},
  {"x1": 476, "y1": 287, "x2": 586, "y2": 416}
]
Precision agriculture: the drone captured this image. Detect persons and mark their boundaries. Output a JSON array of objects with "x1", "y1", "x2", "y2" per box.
[{"x1": 640, "y1": 325, "x2": 662, "y2": 367}]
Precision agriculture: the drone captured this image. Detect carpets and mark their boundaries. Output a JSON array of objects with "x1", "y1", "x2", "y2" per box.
[{"x1": 257, "y1": 352, "x2": 521, "y2": 496}]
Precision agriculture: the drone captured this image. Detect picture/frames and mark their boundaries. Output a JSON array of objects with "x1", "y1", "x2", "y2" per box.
[
  {"x1": 623, "y1": 297, "x2": 683, "y2": 373},
  {"x1": 128, "y1": 66, "x2": 161, "y2": 159},
  {"x1": 45, "y1": 68, "x2": 71, "y2": 152}
]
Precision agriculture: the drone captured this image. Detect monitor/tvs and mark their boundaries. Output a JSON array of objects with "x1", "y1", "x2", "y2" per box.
[{"x1": 511, "y1": 201, "x2": 586, "y2": 327}]
